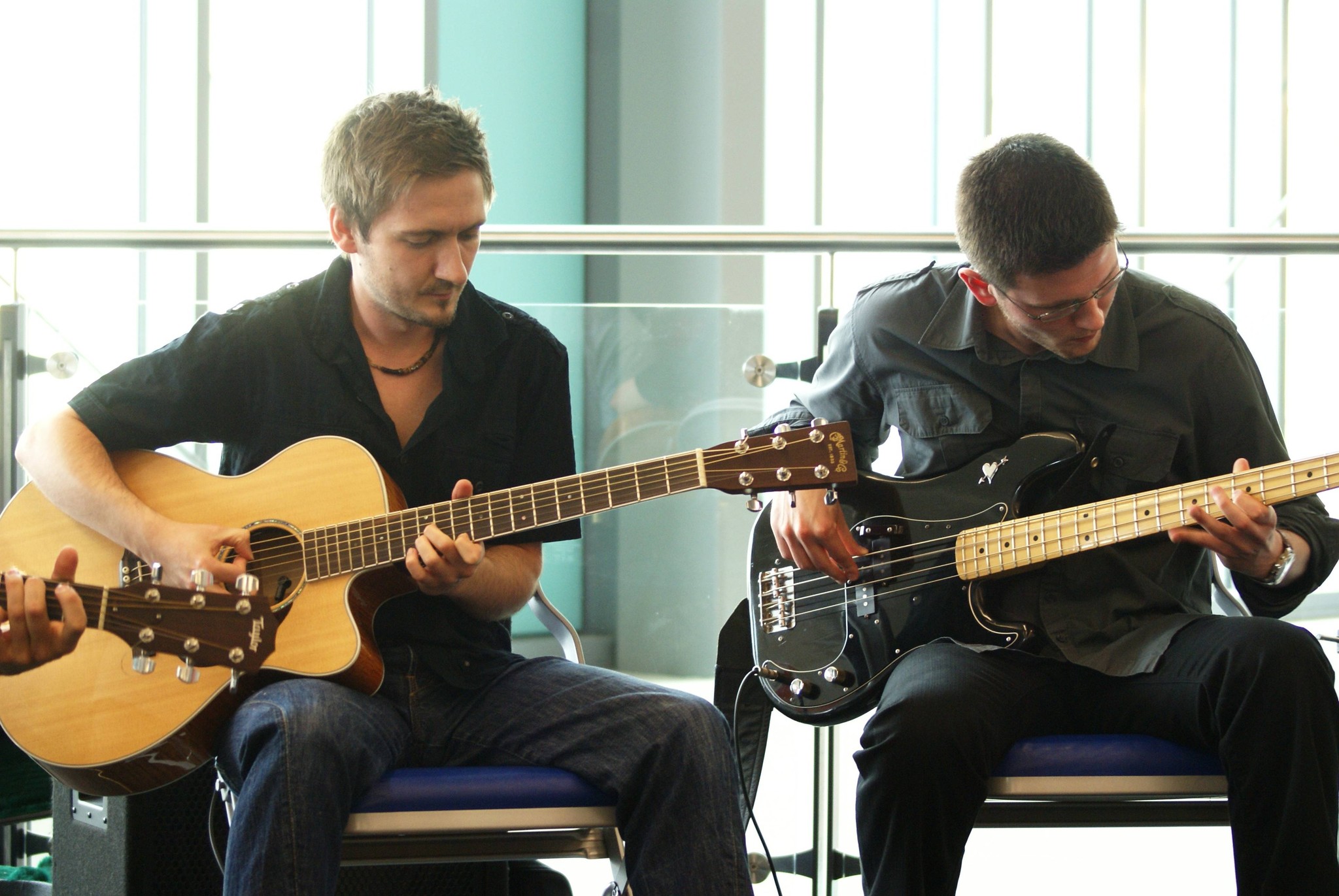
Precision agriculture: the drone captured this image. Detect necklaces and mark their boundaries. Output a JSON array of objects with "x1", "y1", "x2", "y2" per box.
[{"x1": 363, "y1": 327, "x2": 440, "y2": 375}]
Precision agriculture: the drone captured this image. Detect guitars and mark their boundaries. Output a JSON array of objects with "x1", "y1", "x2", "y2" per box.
[
  {"x1": 745, "y1": 432, "x2": 1339, "y2": 727},
  {"x1": 0, "y1": 563, "x2": 276, "y2": 694},
  {"x1": 0, "y1": 412, "x2": 863, "y2": 797}
]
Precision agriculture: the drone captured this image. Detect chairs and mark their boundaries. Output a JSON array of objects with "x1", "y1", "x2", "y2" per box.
[
  {"x1": 970, "y1": 729, "x2": 1237, "y2": 830},
  {"x1": 208, "y1": 580, "x2": 640, "y2": 896}
]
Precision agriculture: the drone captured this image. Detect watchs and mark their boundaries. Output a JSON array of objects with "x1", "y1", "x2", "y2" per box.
[{"x1": 1251, "y1": 527, "x2": 1298, "y2": 588}]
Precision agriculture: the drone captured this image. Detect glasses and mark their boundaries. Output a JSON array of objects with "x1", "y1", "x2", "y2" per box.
[{"x1": 983, "y1": 237, "x2": 1129, "y2": 323}]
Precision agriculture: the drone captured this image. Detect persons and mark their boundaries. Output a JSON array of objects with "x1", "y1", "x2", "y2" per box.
[
  {"x1": 770, "y1": 134, "x2": 1339, "y2": 896},
  {"x1": 0, "y1": 548, "x2": 87, "y2": 677},
  {"x1": 14, "y1": 87, "x2": 755, "y2": 896}
]
